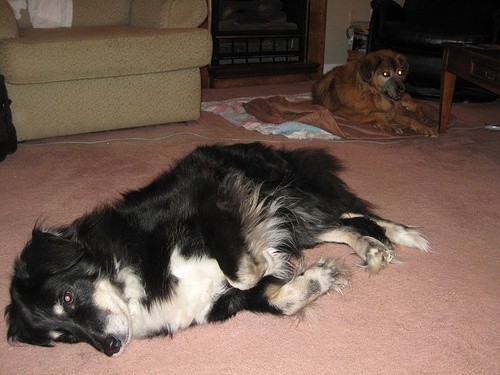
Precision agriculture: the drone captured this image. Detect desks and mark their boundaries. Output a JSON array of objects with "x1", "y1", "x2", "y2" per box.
[{"x1": 437, "y1": 41, "x2": 500, "y2": 136}]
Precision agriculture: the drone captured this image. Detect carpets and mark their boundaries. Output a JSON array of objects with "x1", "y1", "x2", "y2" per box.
[
  {"x1": 198, "y1": 93, "x2": 343, "y2": 140},
  {"x1": 239, "y1": 95, "x2": 457, "y2": 141}
]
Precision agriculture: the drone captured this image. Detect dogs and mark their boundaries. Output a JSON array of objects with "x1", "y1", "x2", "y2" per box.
[
  {"x1": 4, "y1": 140, "x2": 434, "y2": 358},
  {"x1": 311, "y1": 49, "x2": 437, "y2": 137}
]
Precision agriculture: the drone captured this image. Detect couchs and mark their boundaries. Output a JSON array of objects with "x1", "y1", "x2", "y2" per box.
[
  {"x1": 0, "y1": 0, "x2": 214, "y2": 145},
  {"x1": 366, "y1": 0, "x2": 500, "y2": 103}
]
